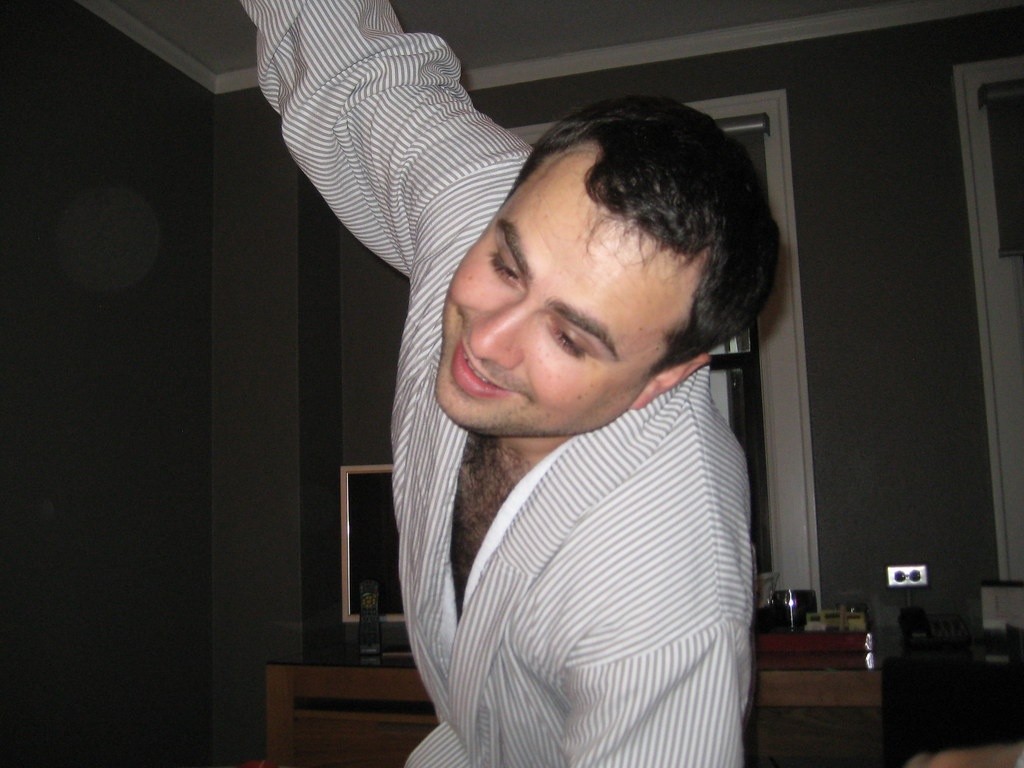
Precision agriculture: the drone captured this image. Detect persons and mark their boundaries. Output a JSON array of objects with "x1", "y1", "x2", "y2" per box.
[{"x1": 237, "y1": 0, "x2": 788, "y2": 768}]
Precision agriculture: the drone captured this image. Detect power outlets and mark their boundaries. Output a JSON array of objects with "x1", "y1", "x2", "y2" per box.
[{"x1": 887, "y1": 565, "x2": 927, "y2": 588}]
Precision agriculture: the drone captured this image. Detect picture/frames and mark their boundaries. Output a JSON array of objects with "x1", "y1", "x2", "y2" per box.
[{"x1": 340, "y1": 464, "x2": 409, "y2": 623}]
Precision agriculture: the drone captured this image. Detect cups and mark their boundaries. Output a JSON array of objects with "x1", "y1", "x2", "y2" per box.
[{"x1": 772, "y1": 590, "x2": 818, "y2": 634}]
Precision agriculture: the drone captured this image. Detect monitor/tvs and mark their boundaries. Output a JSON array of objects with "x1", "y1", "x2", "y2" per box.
[{"x1": 340, "y1": 463, "x2": 406, "y2": 624}]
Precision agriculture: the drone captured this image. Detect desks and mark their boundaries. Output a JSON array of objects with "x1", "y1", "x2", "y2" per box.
[{"x1": 264, "y1": 619, "x2": 888, "y2": 768}]
